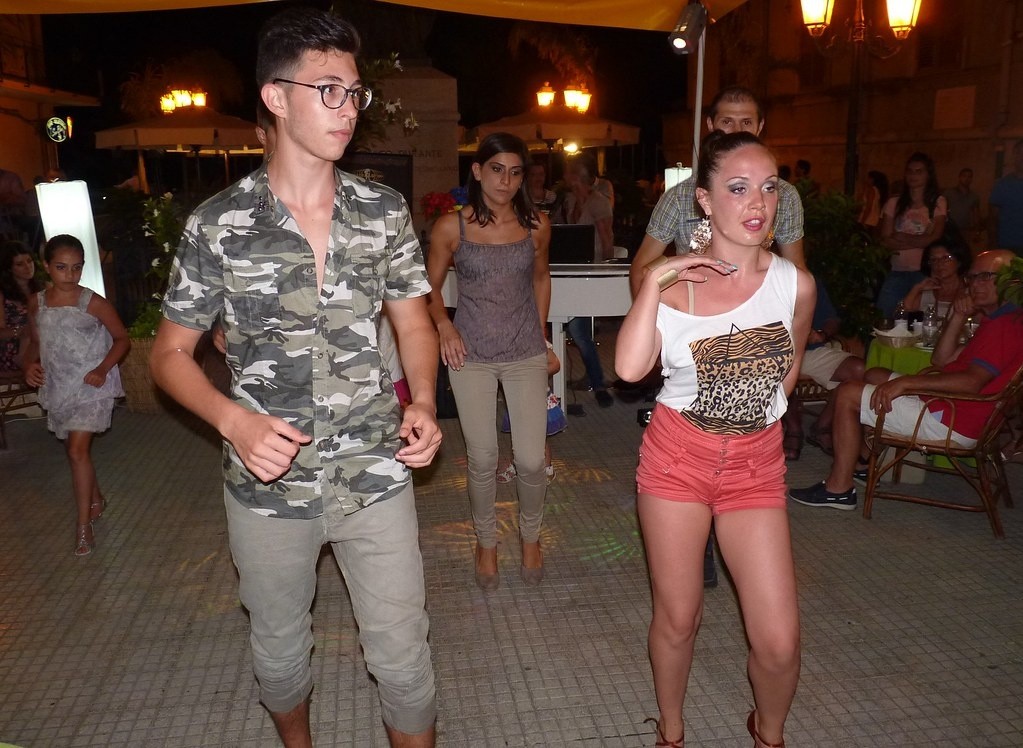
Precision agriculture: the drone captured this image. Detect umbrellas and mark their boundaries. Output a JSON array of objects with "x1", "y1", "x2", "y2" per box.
[
  {"x1": 94, "y1": 106, "x2": 266, "y2": 194},
  {"x1": 478, "y1": 107, "x2": 640, "y2": 151}
]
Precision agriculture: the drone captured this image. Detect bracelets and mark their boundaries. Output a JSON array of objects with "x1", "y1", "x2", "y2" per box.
[{"x1": 642, "y1": 255, "x2": 678, "y2": 292}]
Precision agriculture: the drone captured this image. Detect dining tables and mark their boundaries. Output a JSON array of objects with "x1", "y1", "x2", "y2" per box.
[{"x1": 861, "y1": 328, "x2": 940, "y2": 374}]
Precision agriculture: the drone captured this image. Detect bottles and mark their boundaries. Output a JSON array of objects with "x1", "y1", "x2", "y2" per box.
[
  {"x1": 892, "y1": 300, "x2": 908, "y2": 330},
  {"x1": 922, "y1": 303, "x2": 936, "y2": 347}
]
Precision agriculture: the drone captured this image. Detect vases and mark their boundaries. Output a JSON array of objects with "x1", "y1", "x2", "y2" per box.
[{"x1": 113, "y1": 337, "x2": 180, "y2": 416}]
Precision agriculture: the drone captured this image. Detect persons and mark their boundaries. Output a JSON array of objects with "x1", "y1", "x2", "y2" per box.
[
  {"x1": 777, "y1": 140, "x2": 1023, "y2": 510},
  {"x1": 0, "y1": 241, "x2": 48, "y2": 373},
  {"x1": 21, "y1": 235, "x2": 132, "y2": 556},
  {"x1": 615, "y1": 128, "x2": 817, "y2": 748},
  {"x1": 522, "y1": 152, "x2": 614, "y2": 408},
  {"x1": 0, "y1": 168, "x2": 62, "y2": 253},
  {"x1": 425, "y1": 132, "x2": 551, "y2": 592},
  {"x1": 496, "y1": 338, "x2": 568, "y2": 485},
  {"x1": 148, "y1": 7, "x2": 443, "y2": 748},
  {"x1": 630, "y1": 86, "x2": 805, "y2": 587}
]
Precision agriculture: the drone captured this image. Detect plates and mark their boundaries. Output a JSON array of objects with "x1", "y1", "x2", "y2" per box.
[{"x1": 914, "y1": 342, "x2": 935, "y2": 350}]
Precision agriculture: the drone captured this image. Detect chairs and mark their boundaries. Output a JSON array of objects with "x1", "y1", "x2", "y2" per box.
[
  {"x1": 792, "y1": 332, "x2": 853, "y2": 419},
  {"x1": 0, "y1": 375, "x2": 47, "y2": 449},
  {"x1": 863, "y1": 364, "x2": 1023, "y2": 540}
]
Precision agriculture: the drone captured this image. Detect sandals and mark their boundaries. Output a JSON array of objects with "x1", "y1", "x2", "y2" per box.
[
  {"x1": 495, "y1": 462, "x2": 518, "y2": 483},
  {"x1": 545, "y1": 465, "x2": 557, "y2": 483},
  {"x1": 87, "y1": 498, "x2": 106, "y2": 525},
  {"x1": 75, "y1": 519, "x2": 94, "y2": 556},
  {"x1": 745, "y1": 709, "x2": 787, "y2": 748},
  {"x1": 803, "y1": 421, "x2": 836, "y2": 456},
  {"x1": 644, "y1": 716, "x2": 685, "y2": 748},
  {"x1": 782, "y1": 428, "x2": 805, "y2": 459}
]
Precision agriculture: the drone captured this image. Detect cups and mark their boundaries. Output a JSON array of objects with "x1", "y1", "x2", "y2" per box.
[{"x1": 912, "y1": 322, "x2": 922, "y2": 336}]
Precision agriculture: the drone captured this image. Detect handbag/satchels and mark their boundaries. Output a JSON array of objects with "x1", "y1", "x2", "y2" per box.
[{"x1": 931, "y1": 195, "x2": 970, "y2": 273}]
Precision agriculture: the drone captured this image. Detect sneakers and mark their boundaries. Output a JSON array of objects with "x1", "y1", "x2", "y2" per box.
[
  {"x1": 851, "y1": 468, "x2": 881, "y2": 489},
  {"x1": 789, "y1": 480, "x2": 857, "y2": 510}
]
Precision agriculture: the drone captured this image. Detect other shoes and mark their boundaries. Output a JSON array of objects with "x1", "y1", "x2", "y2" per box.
[
  {"x1": 474, "y1": 542, "x2": 500, "y2": 592},
  {"x1": 518, "y1": 535, "x2": 544, "y2": 585},
  {"x1": 594, "y1": 388, "x2": 614, "y2": 408}
]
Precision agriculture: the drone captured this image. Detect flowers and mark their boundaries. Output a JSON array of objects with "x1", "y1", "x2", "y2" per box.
[
  {"x1": 417, "y1": 186, "x2": 470, "y2": 225},
  {"x1": 125, "y1": 192, "x2": 198, "y2": 338}
]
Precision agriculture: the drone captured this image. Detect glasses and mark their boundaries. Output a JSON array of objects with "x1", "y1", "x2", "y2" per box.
[
  {"x1": 271, "y1": 78, "x2": 373, "y2": 112},
  {"x1": 963, "y1": 272, "x2": 997, "y2": 284},
  {"x1": 928, "y1": 255, "x2": 957, "y2": 265}
]
